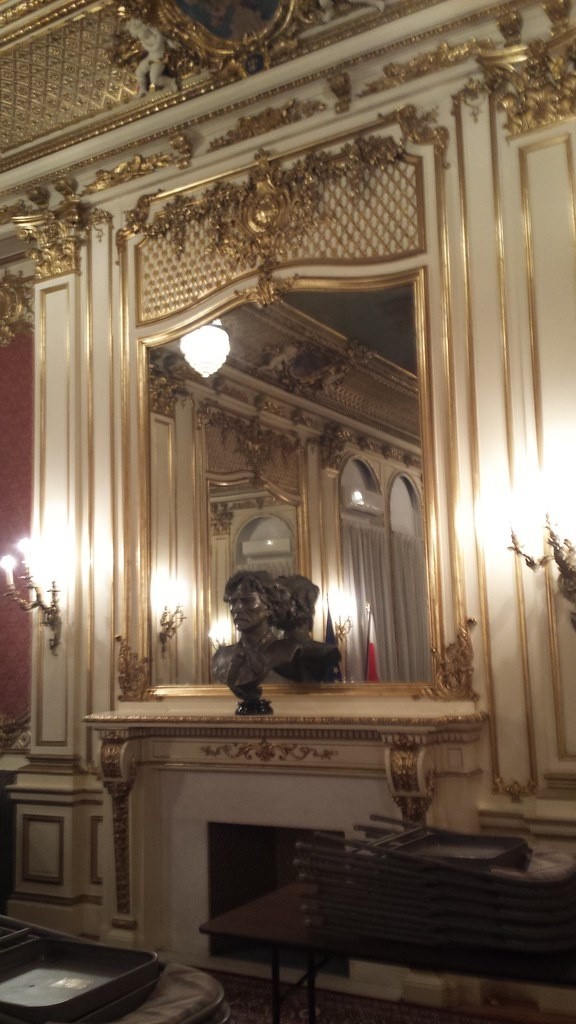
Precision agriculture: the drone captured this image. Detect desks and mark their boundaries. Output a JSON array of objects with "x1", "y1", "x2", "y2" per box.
[
  {"x1": 200, "y1": 882, "x2": 576, "y2": 1024},
  {"x1": 110, "y1": 963, "x2": 231, "y2": 1024}
]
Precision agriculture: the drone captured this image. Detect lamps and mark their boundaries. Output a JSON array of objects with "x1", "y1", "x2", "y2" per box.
[
  {"x1": 0, "y1": 536, "x2": 62, "y2": 657},
  {"x1": 503, "y1": 471, "x2": 576, "y2": 626},
  {"x1": 180, "y1": 317, "x2": 231, "y2": 378}
]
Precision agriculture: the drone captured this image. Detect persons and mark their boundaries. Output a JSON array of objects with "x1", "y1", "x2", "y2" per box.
[
  {"x1": 274, "y1": 573, "x2": 342, "y2": 683},
  {"x1": 208, "y1": 570, "x2": 308, "y2": 685}
]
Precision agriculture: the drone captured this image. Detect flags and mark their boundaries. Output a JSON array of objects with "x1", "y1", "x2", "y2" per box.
[
  {"x1": 326, "y1": 608, "x2": 342, "y2": 682},
  {"x1": 368, "y1": 614, "x2": 381, "y2": 683}
]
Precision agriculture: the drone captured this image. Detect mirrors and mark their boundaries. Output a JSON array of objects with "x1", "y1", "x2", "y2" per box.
[{"x1": 116, "y1": 266, "x2": 448, "y2": 699}]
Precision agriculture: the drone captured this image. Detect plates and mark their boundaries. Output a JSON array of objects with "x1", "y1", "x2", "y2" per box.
[{"x1": 0, "y1": 912, "x2": 230, "y2": 1024}]
